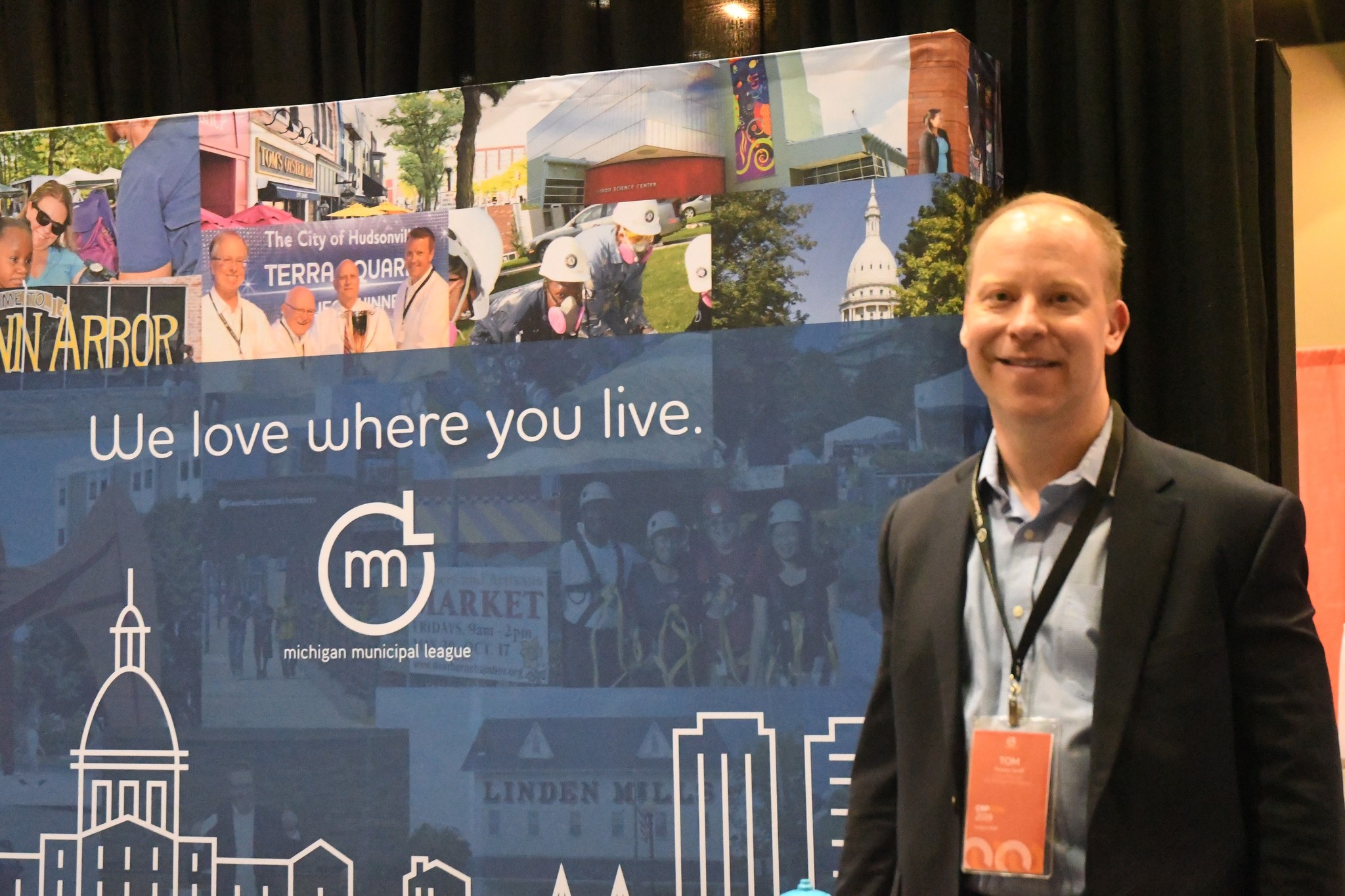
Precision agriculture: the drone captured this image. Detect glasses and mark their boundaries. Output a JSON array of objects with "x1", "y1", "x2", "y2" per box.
[
  {"x1": 210, "y1": 254, "x2": 249, "y2": 269},
  {"x1": 284, "y1": 303, "x2": 317, "y2": 316},
  {"x1": 449, "y1": 275, "x2": 474, "y2": 322},
  {"x1": 32, "y1": 201, "x2": 67, "y2": 235}
]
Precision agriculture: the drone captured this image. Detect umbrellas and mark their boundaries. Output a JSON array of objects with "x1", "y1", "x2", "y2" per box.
[
  {"x1": 201, "y1": 208, "x2": 242, "y2": 231},
  {"x1": 327, "y1": 203, "x2": 386, "y2": 218},
  {"x1": 369, "y1": 202, "x2": 412, "y2": 214},
  {"x1": 226, "y1": 202, "x2": 302, "y2": 226}
]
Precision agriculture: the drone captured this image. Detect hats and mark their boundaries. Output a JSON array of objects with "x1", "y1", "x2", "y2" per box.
[{"x1": 104, "y1": 123, "x2": 120, "y2": 144}]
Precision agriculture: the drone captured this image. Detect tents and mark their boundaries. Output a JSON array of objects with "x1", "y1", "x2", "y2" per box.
[
  {"x1": 914, "y1": 363, "x2": 988, "y2": 452},
  {"x1": 55, "y1": 167, "x2": 117, "y2": 202},
  {"x1": 97, "y1": 167, "x2": 122, "y2": 179},
  {"x1": 823, "y1": 416, "x2": 907, "y2": 461}
]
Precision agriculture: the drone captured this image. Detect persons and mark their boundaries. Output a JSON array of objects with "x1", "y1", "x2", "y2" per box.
[
  {"x1": 449, "y1": 199, "x2": 712, "y2": 346},
  {"x1": 918, "y1": 109, "x2": 954, "y2": 174},
  {"x1": 834, "y1": 452, "x2": 876, "y2": 506},
  {"x1": 18, "y1": 696, "x2": 47, "y2": 785},
  {"x1": 1, "y1": 115, "x2": 200, "y2": 287},
  {"x1": 559, "y1": 481, "x2": 838, "y2": 688},
  {"x1": 208, "y1": 764, "x2": 300, "y2": 896},
  {"x1": 228, "y1": 595, "x2": 298, "y2": 680},
  {"x1": 832, "y1": 192, "x2": 1345, "y2": 896},
  {"x1": 202, "y1": 227, "x2": 449, "y2": 414}
]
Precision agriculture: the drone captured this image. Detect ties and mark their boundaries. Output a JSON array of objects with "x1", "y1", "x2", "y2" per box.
[{"x1": 344, "y1": 311, "x2": 353, "y2": 354}]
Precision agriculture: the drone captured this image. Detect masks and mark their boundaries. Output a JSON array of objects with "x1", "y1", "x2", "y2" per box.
[{"x1": 699, "y1": 291, "x2": 714, "y2": 309}]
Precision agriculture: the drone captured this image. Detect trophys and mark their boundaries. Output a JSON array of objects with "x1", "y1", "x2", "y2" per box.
[{"x1": 341, "y1": 310, "x2": 376, "y2": 385}]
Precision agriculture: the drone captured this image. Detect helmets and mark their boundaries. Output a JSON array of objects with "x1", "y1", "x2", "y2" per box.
[
  {"x1": 702, "y1": 489, "x2": 741, "y2": 524},
  {"x1": 612, "y1": 198, "x2": 662, "y2": 236},
  {"x1": 684, "y1": 234, "x2": 712, "y2": 292},
  {"x1": 768, "y1": 499, "x2": 807, "y2": 529},
  {"x1": 447, "y1": 207, "x2": 503, "y2": 319},
  {"x1": 539, "y1": 237, "x2": 591, "y2": 283},
  {"x1": 648, "y1": 511, "x2": 684, "y2": 537},
  {"x1": 579, "y1": 481, "x2": 616, "y2": 512}
]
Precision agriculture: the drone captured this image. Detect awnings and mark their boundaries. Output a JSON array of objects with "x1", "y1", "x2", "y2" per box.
[
  {"x1": 262, "y1": 181, "x2": 320, "y2": 201},
  {"x1": 363, "y1": 174, "x2": 387, "y2": 197}
]
